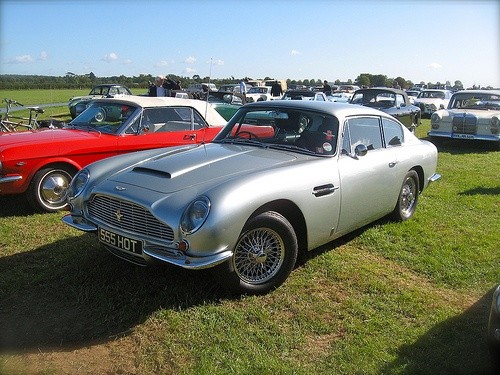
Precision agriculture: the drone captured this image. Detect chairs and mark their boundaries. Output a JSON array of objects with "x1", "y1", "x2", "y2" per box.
[
  {"x1": 334, "y1": 89, "x2": 340, "y2": 93},
  {"x1": 255, "y1": 88, "x2": 259, "y2": 93},
  {"x1": 348, "y1": 89, "x2": 352, "y2": 93},
  {"x1": 318, "y1": 117, "x2": 339, "y2": 155},
  {"x1": 341, "y1": 89, "x2": 347, "y2": 93},
  {"x1": 426, "y1": 92, "x2": 431, "y2": 98},
  {"x1": 295, "y1": 130, "x2": 324, "y2": 152},
  {"x1": 379, "y1": 100, "x2": 391, "y2": 104},
  {"x1": 434, "y1": 92, "x2": 445, "y2": 99}
]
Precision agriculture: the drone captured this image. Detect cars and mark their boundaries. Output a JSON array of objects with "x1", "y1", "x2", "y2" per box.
[
  {"x1": 0, "y1": 97, "x2": 275, "y2": 212},
  {"x1": 60, "y1": 100, "x2": 442, "y2": 295},
  {"x1": 67, "y1": 83, "x2": 134, "y2": 120},
  {"x1": 166, "y1": 79, "x2": 500, "y2": 151}
]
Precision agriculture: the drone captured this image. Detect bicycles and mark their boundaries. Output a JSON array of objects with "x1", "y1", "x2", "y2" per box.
[{"x1": 0, "y1": 97, "x2": 68, "y2": 132}]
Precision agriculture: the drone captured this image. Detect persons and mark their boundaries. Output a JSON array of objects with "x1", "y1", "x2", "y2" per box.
[
  {"x1": 149, "y1": 75, "x2": 177, "y2": 97},
  {"x1": 271, "y1": 81, "x2": 283, "y2": 99},
  {"x1": 323, "y1": 81, "x2": 332, "y2": 99},
  {"x1": 240, "y1": 79, "x2": 248, "y2": 98},
  {"x1": 176, "y1": 80, "x2": 181, "y2": 90}
]
